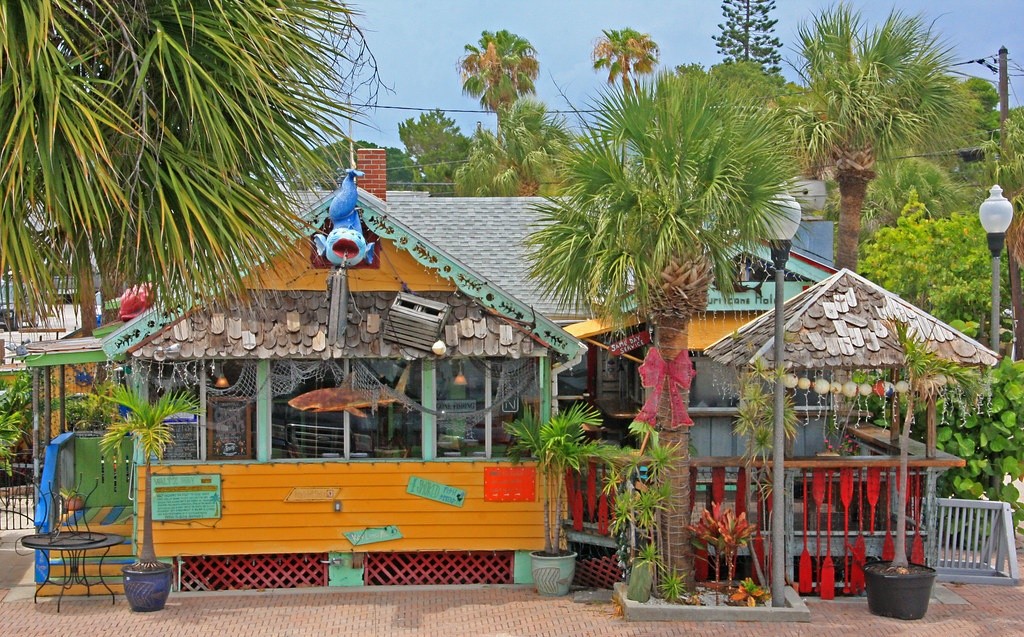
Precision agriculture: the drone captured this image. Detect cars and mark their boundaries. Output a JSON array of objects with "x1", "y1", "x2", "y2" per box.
[
  {"x1": 0, "y1": 281, "x2": 39, "y2": 331},
  {"x1": 46, "y1": 264, "x2": 101, "y2": 301}
]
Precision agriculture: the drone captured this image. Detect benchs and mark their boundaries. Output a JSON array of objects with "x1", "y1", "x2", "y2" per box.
[{"x1": 696, "y1": 478, "x2": 767, "y2": 531}]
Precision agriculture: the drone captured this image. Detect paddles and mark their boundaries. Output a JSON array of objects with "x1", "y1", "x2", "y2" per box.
[
  {"x1": 812, "y1": 468, "x2": 826, "y2": 592},
  {"x1": 799, "y1": 469, "x2": 813, "y2": 594},
  {"x1": 867, "y1": 467, "x2": 926, "y2": 566},
  {"x1": 820, "y1": 468, "x2": 835, "y2": 600},
  {"x1": 839, "y1": 467, "x2": 854, "y2": 594},
  {"x1": 565, "y1": 457, "x2": 617, "y2": 535},
  {"x1": 688, "y1": 466, "x2": 772, "y2": 585},
  {"x1": 851, "y1": 466, "x2": 865, "y2": 595}
]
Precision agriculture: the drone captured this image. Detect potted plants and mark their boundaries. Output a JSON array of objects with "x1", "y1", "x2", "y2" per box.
[
  {"x1": 862, "y1": 313, "x2": 984, "y2": 621},
  {"x1": 500, "y1": 399, "x2": 603, "y2": 598},
  {"x1": 98, "y1": 379, "x2": 207, "y2": 612}
]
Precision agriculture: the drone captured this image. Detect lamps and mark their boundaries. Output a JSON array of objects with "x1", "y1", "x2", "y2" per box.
[
  {"x1": 454, "y1": 360, "x2": 469, "y2": 387},
  {"x1": 215, "y1": 362, "x2": 230, "y2": 388}
]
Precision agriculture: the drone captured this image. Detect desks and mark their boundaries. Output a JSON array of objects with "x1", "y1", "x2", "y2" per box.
[
  {"x1": 20, "y1": 472, "x2": 107, "y2": 547},
  {"x1": 21, "y1": 531, "x2": 124, "y2": 613}
]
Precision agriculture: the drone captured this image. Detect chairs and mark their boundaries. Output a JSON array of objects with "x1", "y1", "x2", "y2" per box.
[{"x1": 285, "y1": 423, "x2": 354, "y2": 459}]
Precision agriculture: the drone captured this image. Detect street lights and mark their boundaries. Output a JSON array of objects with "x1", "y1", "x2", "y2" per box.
[
  {"x1": 978, "y1": 184, "x2": 1014, "y2": 356},
  {"x1": 757, "y1": 186, "x2": 803, "y2": 613}
]
